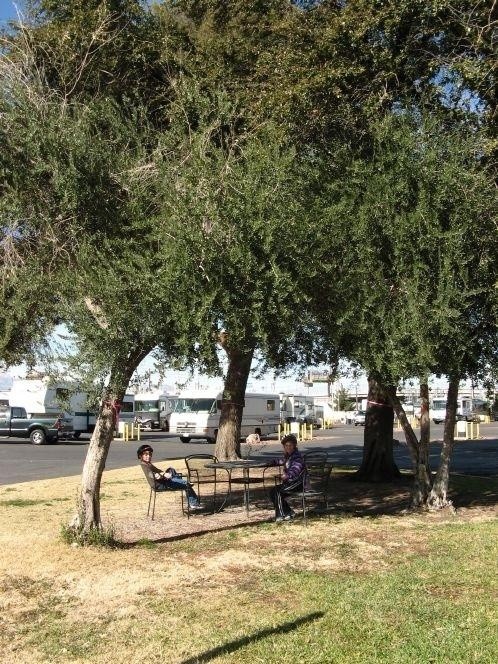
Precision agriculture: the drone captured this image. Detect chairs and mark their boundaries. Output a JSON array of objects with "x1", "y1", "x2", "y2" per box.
[
  {"x1": 184, "y1": 454, "x2": 229, "y2": 504},
  {"x1": 140, "y1": 463, "x2": 190, "y2": 519},
  {"x1": 290, "y1": 464, "x2": 334, "y2": 520},
  {"x1": 274, "y1": 449, "x2": 329, "y2": 488}
]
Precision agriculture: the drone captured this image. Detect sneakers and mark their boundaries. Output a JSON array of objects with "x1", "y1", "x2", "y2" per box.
[
  {"x1": 284, "y1": 514, "x2": 295, "y2": 521},
  {"x1": 275, "y1": 517, "x2": 283, "y2": 523}
]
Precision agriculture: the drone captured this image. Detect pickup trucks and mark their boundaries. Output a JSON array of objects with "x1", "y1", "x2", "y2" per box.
[{"x1": 0, "y1": 406, "x2": 74, "y2": 445}]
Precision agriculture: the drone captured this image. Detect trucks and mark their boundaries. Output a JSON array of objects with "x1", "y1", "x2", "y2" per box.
[
  {"x1": 134, "y1": 391, "x2": 179, "y2": 430},
  {"x1": 278, "y1": 393, "x2": 323, "y2": 430},
  {"x1": 393, "y1": 402, "x2": 421, "y2": 423},
  {"x1": 429, "y1": 399, "x2": 473, "y2": 425},
  {"x1": 169, "y1": 390, "x2": 281, "y2": 443}
]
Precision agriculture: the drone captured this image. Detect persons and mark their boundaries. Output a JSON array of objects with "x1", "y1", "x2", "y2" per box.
[
  {"x1": 136, "y1": 444, "x2": 213, "y2": 516},
  {"x1": 269, "y1": 434, "x2": 313, "y2": 522}
]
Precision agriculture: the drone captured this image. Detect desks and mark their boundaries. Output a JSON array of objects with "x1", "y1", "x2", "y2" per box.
[{"x1": 204, "y1": 459, "x2": 272, "y2": 518}]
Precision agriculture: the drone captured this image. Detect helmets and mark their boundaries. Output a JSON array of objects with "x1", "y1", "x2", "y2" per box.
[{"x1": 137, "y1": 445, "x2": 153, "y2": 459}]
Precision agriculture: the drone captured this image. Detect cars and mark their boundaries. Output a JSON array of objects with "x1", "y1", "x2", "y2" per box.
[{"x1": 353, "y1": 410, "x2": 366, "y2": 425}]
